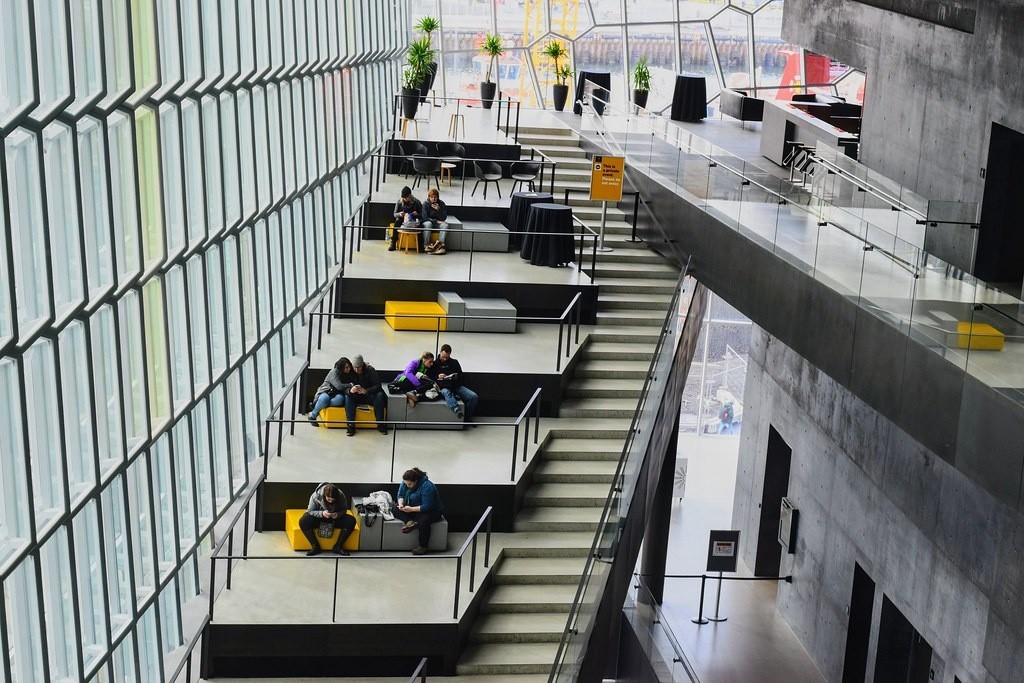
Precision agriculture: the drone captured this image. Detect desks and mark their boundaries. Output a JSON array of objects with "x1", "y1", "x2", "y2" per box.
[
  {"x1": 440, "y1": 163, "x2": 456, "y2": 186},
  {"x1": 397, "y1": 230, "x2": 421, "y2": 254},
  {"x1": 507, "y1": 192, "x2": 553, "y2": 252},
  {"x1": 523, "y1": 206, "x2": 576, "y2": 268},
  {"x1": 574, "y1": 68, "x2": 611, "y2": 116},
  {"x1": 671, "y1": 74, "x2": 707, "y2": 123}
]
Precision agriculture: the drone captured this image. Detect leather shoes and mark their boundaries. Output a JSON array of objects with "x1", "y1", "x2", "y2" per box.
[
  {"x1": 332, "y1": 545, "x2": 350, "y2": 556},
  {"x1": 306, "y1": 544, "x2": 322, "y2": 555}
]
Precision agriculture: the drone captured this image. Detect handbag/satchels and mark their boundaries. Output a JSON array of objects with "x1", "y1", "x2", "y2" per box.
[
  {"x1": 400, "y1": 214, "x2": 420, "y2": 232},
  {"x1": 355, "y1": 503, "x2": 379, "y2": 527}
]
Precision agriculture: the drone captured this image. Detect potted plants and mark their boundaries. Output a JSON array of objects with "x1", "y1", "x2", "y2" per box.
[
  {"x1": 630, "y1": 55, "x2": 654, "y2": 112},
  {"x1": 400, "y1": 17, "x2": 438, "y2": 118},
  {"x1": 480, "y1": 31, "x2": 503, "y2": 109},
  {"x1": 539, "y1": 41, "x2": 572, "y2": 112}
]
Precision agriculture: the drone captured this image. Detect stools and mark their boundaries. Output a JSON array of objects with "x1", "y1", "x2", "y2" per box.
[
  {"x1": 402, "y1": 118, "x2": 418, "y2": 138},
  {"x1": 448, "y1": 113, "x2": 465, "y2": 139},
  {"x1": 782, "y1": 140, "x2": 820, "y2": 191}
]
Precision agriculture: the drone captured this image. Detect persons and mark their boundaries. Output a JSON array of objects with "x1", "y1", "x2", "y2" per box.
[
  {"x1": 344, "y1": 355, "x2": 388, "y2": 436},
  {"x1": 420, "y1": 189, "x2": 449, "y2": 255},
  {"x1": 391, "y1": 468, "x2": 444, "y2": 555},
  {"x1": 299, "y1": 482, "x2": 356, "y2": 555},
  {"x1": 392, "y1": 352, "x2": 440, "y2": 408},
  {"x1": 308, "y1": 357, "x2": 352, "y2": 427},
  {"x1": 718, "y1": 401, "x2": 733, "y2": 435},
  {"x1": 387, "y1": 186, "x2": 422, "y2": 251},
  {"x1": 428, "y1": 344, "x2": 479, "y2": 427}
]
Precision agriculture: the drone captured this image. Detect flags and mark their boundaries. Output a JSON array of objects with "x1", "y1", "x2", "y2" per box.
[{"x1": 775, "y1": 48, "x2": 831, "y2": 102}]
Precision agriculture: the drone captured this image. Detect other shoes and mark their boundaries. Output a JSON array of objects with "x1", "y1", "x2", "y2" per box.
[
  {"x1": 464, "y1": 418, "x2": 477, "y2": 428},
  {"x1": 308, "y1": 416, "x2": 319, "y2": 427},
  {"x1": 412, "y1": 546, "x2": 429, "y2": 555},
  {"x1": 346, "y1": 423, "x2": 356, "y2": 435},
  {"x1": 406, "y1": 390, "x2": 417, "y2": 401},
  {"x1": 407, "y1": 398, "x2": 415, "y2": 407},
  {"x1": 388, "y1": 244, "x2": 396, "y2": 251},
  {"x1": 377, "y1": 424, "x2": 387, "y2": 434},
  {"x1": 454, "y1": 407, "x2": 463, "y2": 418},
  {"x1": 401, "y1": 520, "x2": 419, "y2": 533}
]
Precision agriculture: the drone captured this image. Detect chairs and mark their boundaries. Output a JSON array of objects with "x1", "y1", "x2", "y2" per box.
[
  {"x1": 398, "y1": 141, "x2": 427, "y2": 179},
  {"x1": 509, "y1": 159, "x2": 540, "y2": 197},
  {"x1": 412, "y1": 154, "x2": 441, "y2": 190},
  {"x1": 436, "y1": 142, "x2": 466, "y2": 181},
  {"x1": 472, "y1": 160, "x2": 502, "y2": 199}
]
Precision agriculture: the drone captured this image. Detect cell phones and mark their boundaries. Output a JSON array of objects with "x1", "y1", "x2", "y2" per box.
[
  {"x1": 355, "y1": 385, "x2": 360, "y2": 388},
  {"x1": 330, "y1": 513, "x2": 335, "y2": 515}
]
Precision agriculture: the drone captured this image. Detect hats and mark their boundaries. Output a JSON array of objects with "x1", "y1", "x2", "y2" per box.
[
  {"x1": 401, "y1": 185, "x2": 411, "y2": 198},
  {"x1": 352, "y1": 355, "x2": 363, "y2": 364}
]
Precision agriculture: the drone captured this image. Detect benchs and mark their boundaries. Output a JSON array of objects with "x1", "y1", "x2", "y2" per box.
[
  {"x1": 464, "y1": 298, "x2": 517, "y2": 333},
  {"x1": 384, "y1": 300, "x2": 446, "y2": 330},
  {"x1": 389, "y1": 223, "x2": 439, "y2": 248},
  {"x1": 791, "y1": 93, "x2": 846, "y2": 104},
  {"x1": 317, "y1": 406, "x2": 386, "y2": 428},
  {"x1": 898, "y1": 315, "x2": 943, "y2": 347},
  {"x1": 285, "y1": 507, "x2": 360, "y2": 552},
  {"x1": 461, "y1": 221, "x2": 509, "y2": 252},
  {"x1": 406, "y1": 401, "x2": 465, "y2": 432},
  {"x1": 382, "y1": 517, "x2": 448, "y2": 552},
  {"x1": 720, "y1": 89, "x2": 764, "y2": 130},
  {"x1": 958, "y1": 322, "x2": 1006, "y2": 351}
]
